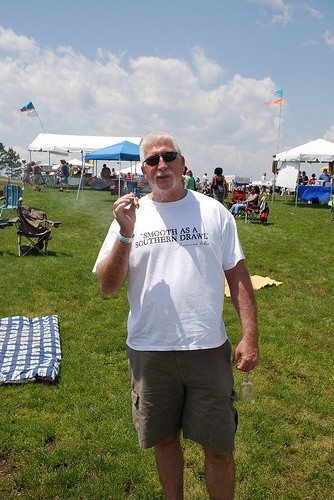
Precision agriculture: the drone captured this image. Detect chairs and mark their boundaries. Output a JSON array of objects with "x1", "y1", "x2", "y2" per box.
[
  {"x1": 239, "y1": 196, "x2": 267, "y2": 224},
  {"x1": 7, "y1": 205, "x2": 61, "y2": 258},
  {"x1": 0, "y1": 184, "x2": 23, "y2": 214}
]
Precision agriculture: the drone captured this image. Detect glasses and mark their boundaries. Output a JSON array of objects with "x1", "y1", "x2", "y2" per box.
[{"x1": 143, "y1": 151, "x2": 179, "y2": 166}]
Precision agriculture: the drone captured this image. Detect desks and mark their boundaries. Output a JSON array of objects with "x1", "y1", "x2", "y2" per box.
[
  {"x1": 68, "y1": 178, "x2": 122, "y2": 189},
  {"x1": 298, "y1": 185, "x2": 334, "y2": 205}
]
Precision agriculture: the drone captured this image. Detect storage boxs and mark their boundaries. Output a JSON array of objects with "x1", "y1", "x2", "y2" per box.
[{"x1": 315, "y1": 180, "x2": 325, "y2": 186}]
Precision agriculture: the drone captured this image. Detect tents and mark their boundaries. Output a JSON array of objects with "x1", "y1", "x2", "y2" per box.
[
  {"x1": 116, "y1": 163, "x2": 143, "y2": 176},
  {"x1": 76, "y1": 140, "x2": 140, "y2": 200},
  {"x1": 28, "y1": 133, "x2": 142, "y2": 167},
  {"x1": 272, "y1": 138, "x2": 334, "y2": 208}
]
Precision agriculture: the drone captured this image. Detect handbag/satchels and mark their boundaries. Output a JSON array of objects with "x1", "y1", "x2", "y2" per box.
[{"x1": 18, "y1": 207, "x2": 51, "y2": 233}]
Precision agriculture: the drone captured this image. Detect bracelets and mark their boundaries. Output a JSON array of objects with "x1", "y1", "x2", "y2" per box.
[{"x1": 116, "y1": 230, "x2": 135, "y2": 243}]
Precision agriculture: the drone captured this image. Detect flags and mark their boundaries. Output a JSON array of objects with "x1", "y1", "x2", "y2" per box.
[
  {"x1": 264, "y1": 98, "x2": 283, "y2": 107},
  {"x1": 19, "y1": 101, "x2": 39, "y2": 118},
  {"x1": 273, "y1": 89, "x2": 284, "y2": 97}
]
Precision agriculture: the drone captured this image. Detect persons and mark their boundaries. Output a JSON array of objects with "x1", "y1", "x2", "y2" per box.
[
  {"x1": 21, "y1": 159, "x2": 34, "y2": 191},
  {"x1": 45, "y1": 158, "x2": 81, "y2": 193},
  {"x1": 100, "y1": 164, "x2": 143, "y2": 181},
  {"x1": 30, "y1": 160, "x2": 41, "y2": 192},
  {"x1": 91, "y1": 132, "x2": 260, "y2": 500},
  {"x1": 182, "y1": 165, "x2": 258, "y2": 218},
  {"x1": 261, "y1": 172, "x2": 266, "y2": 194},
  {"x1": 298, "y1": 169, "x2": 331, "y2": 186}
]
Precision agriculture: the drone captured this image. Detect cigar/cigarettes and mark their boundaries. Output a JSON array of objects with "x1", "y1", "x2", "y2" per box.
[{"x1": 130, "y1": 192, "x2": 139, "y2": 209}]
